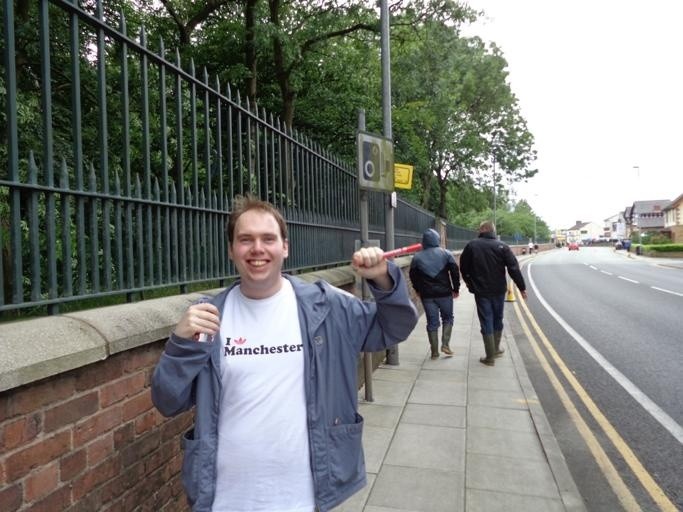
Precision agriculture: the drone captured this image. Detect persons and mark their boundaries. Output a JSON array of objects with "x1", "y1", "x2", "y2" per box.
[
  {"x1": 535, "y1": 244, "x2": 539, "y2": 253},
  {"x1": 409, "y1": 227, "x2": 461, "y2": 360},
  {"x1": 623, "y1": 238, "x2": 631, "y2": 253},
  {"x1": 459, "y1": 220, "x2": 528, "y2": 366},
  {"x1": 149, "y1": 191, "x2": 420, "y2": 512},
  {"x1": 529, "y1": 241, "x2": 533, "y2": 255}
]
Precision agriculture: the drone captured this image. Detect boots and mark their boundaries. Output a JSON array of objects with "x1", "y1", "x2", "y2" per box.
[
  {"x1": 439, "y1": 322, "x2": 454, "y2": 356},
  {"x1": 425, "y1": 329, "x2": 440, "y2": 360},
  {"x1": 478, "y1": 330, "x2": 505, "y2": 367}
]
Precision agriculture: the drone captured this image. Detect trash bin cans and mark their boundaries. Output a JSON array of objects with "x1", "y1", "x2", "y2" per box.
[{"x1": 637, "y1": 246, "x2": 641, "y2": 255}]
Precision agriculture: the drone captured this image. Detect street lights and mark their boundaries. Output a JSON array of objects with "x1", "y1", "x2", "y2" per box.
[{"x1": 630, "y1": 164, "x2": 644, "y2": 257}]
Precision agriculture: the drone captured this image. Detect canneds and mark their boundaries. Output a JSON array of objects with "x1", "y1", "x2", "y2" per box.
[{"x1": 193, "y1": 301, "x2": 214, "y2": 342}]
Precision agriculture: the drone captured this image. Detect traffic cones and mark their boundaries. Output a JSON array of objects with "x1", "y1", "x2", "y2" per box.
[{"x1": 503, "y1": 281, "x2": 516, "y2": 302}]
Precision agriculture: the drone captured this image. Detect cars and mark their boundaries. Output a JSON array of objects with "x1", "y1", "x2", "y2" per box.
[{"x1": 568, "y1": 243, "x2": 579, "y2": 251}]
